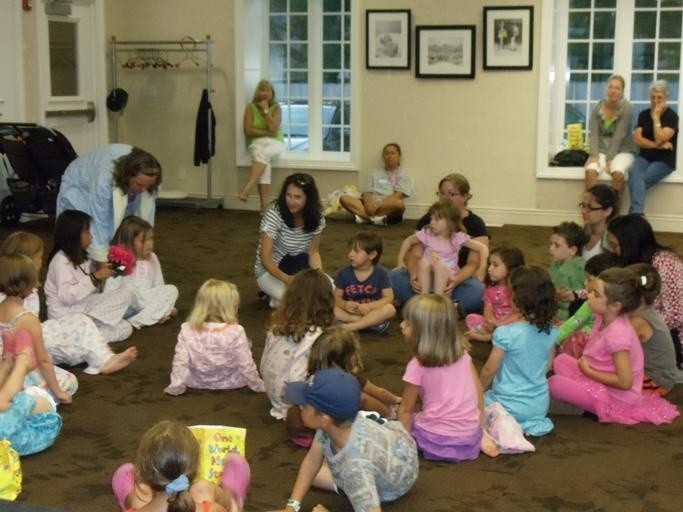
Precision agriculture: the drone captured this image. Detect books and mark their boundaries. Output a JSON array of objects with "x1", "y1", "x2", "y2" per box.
[{"x1": 187, "y1": 424, "x2": 246, "y2": 485}]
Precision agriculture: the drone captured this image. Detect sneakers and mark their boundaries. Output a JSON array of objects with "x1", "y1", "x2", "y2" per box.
[
  {"x1": 354, "y1": 214, "x2": 366, "y2": 224},
  {"x1": 374, "y1": 215, "x2": 387, "y2": 226}
]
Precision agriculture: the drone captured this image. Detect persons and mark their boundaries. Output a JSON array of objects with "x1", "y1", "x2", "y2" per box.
[
  {"x1": 55, "y1": 144, "x2": 162, "y2": 293},
  {"x1": 252, "y1": 173, "x2": 334, "y2": 310},
  {"x1": 109, "y1": 418, "x2": 250, "y2": 511},
  {"x1": 510, "y1": 24, "x2": 519, "y2": 49},
  {"x1": 625, "y1": 80, "x2": 678, "y2": 215},
  {"x1": 162, "y1": 278, "x2": 266, "y2": 397},
  {"x1": 584, "y1": 75, "x2": 636, "y2": 209},
  {"x1": 277, "y1": 370, "x2": 420, "y2": 512},
  {"x1": 0, "y1": 210, "x2": 179, "y2": 502},
  {"x1": 339, "y1": 143, "x2": 410, "y2": 226},
  {"x1": 496, "y1": 21, "x2": 508, "y2": 50},
  {"x1": 255, "y1": 175, "x2": 683, "y2": 463},
  {"x1": 239, "y1": 78, "x2": 284, "y2": 217}
]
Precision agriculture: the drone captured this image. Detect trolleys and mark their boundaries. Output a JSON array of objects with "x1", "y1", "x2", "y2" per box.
[{"x1": 0, "y1": 121, "x2": 79, "y2": 229}]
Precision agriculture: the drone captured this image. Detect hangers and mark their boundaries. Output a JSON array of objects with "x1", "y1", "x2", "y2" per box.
[{"x1": 122, "y1": 48, "x2": 205, "y2": 70}]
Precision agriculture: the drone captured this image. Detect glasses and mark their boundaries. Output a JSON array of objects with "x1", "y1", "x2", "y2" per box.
[
  {"x1": 436, "y1": 192, "x2": 460, "y2": 197},
  {"x1": 287, "y1": 176, "x2": 308, "y2": 186},
  {"x1": 578, "y1": 203, "x2": 603, "y2": 210}
]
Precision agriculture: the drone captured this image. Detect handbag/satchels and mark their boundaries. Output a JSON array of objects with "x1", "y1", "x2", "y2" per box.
[{"x1": 549, "y1": 149, "x2": 589, "y2": 166}]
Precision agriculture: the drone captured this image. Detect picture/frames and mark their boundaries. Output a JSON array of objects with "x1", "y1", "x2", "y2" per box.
[
  {"x1": 365, "y1": 8, "x2": 414, "y2": 74},
  {"x1": 481, "y1": 4, "x2": 537, "y2": 73},
  {"x1": 415, "y1": 23, "x2": 477, "y2": 80}
]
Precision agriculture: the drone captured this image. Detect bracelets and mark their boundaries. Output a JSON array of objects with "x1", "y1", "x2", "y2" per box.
[
  {"x1": 91, "y1": 273, "x2": 98, "y2": 281},
  {"x1": 653, "y1": 123, "x2": 661, "y2": 128},
  {"x1": 286, "y1": 499, "x2": 300, "y2": 511},
  {"x1": 260, "y1": 109, "x2": 269, "y2": 114}
]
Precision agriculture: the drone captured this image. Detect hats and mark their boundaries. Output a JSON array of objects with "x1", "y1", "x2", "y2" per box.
[{"x1": 284, "y1": 369, "x2": 360, "y2": 420}]
